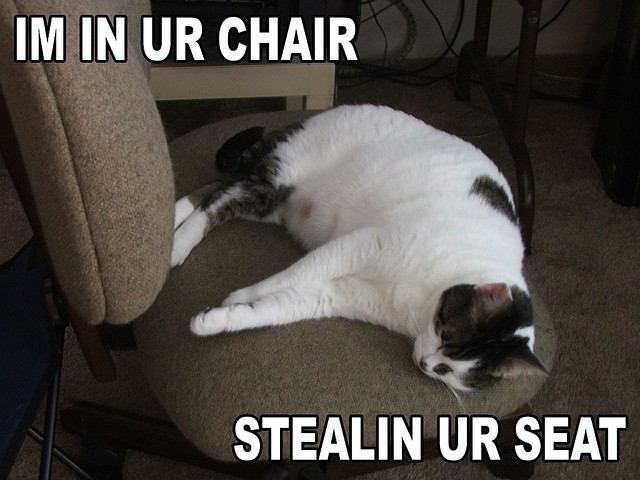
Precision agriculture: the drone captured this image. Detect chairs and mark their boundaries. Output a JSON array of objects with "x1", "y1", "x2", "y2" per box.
[{"x1": 0, "y1": 0, "x2": 559, "y2": 478}]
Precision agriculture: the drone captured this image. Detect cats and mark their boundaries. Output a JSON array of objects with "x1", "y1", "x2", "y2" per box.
[{"x1": 171, "y1": 102, "x2": 550, "y2": 413}]
